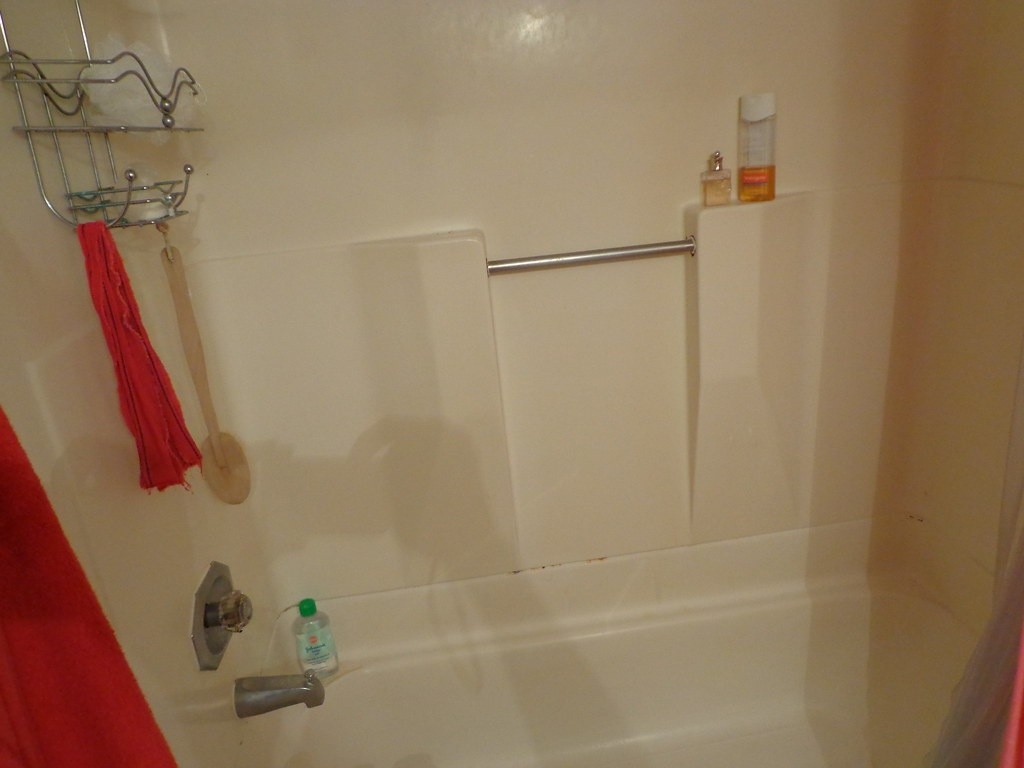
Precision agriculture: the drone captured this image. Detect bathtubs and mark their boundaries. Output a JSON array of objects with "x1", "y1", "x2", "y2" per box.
[{"x1": 224, "y1": 551, "x2": 1006, "y2": 768}]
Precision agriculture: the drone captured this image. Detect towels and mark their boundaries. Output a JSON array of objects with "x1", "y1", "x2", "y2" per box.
[
  {"x1": 0, "y1": 404, "x2": 181, "y2": 768},
  {"x1": 75, "y1": 217, "x2": 205, "y2": 493}
]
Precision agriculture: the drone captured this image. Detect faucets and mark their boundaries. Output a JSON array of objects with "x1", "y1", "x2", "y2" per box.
[{"x1": 232, "y1": 673, "x2": 330, "y2": 720}]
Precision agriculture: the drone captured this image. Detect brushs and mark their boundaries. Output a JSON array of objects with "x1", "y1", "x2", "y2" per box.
[{"x1": 154, "y1": 220, "x2": 251, "y2": 506}]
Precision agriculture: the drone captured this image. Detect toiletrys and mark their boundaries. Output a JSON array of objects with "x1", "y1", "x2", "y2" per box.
[
  {"x1": 734, "y1": 92, "x2": 779, "y2": 204},
  {"x1": 701, "y1": 150, "x2": 736, "y2": 208},
  {"x1": 278, "y1": 596, "x2": 342, "y2": 679}
]
